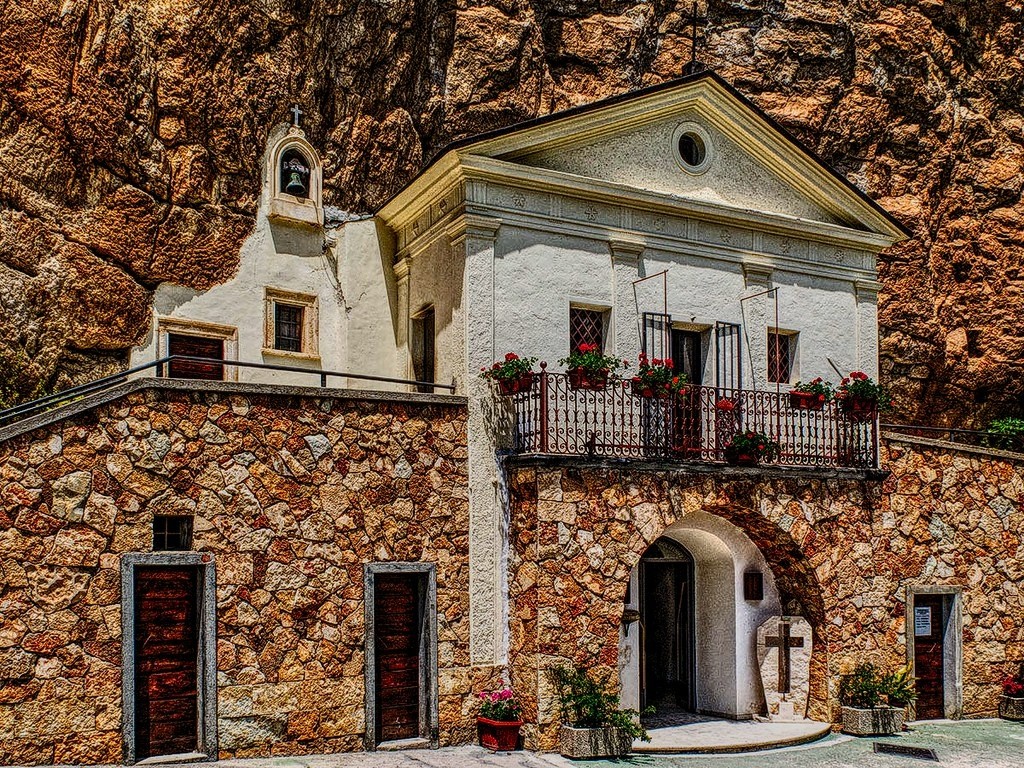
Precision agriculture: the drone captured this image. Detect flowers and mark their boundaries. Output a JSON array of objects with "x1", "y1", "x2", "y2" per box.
[
  {"x1": 633, "y1": 353, "x2": 688, "y2": 400},
  {"x1": 465, "y1": 675, "x2": 521, "y2": 723},
  {"x1": 721, "y1": 431, "x2": 785, "y2": 463},
  {"x1": 834, "y1": 371, "x2": 897, "y2": 414},
  {"x1": 794, "y1": 376, "x2": 835, "y2": 405},
  {"x1": 557, "y1": 342, "x2": 630, "y2": 387},
  {"x1": 1001, "y1": 676, "x2": 1024, "y2": 698},
  {"x1": 478, "y1": 351, "x2": 538, "y2": 383}
]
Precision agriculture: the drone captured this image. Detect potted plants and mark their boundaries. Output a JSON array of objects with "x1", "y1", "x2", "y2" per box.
[
  {"x1": 838, "y1": 659, "x2": 922, "y2": 738},
  {"x1": 544, "y1": 663, "x2": 657, "y2": 762}
]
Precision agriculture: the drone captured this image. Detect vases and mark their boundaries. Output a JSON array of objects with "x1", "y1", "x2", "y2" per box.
[
  {"x1": 477, "y1": 370, "x2": 875, "y2": 466},
  {"x1": 999, "y1": 694, "x2": 1024, "y2": 722},
  {"x1": 477, "y1": 717, "x2": 524, "y2": 751}
]
